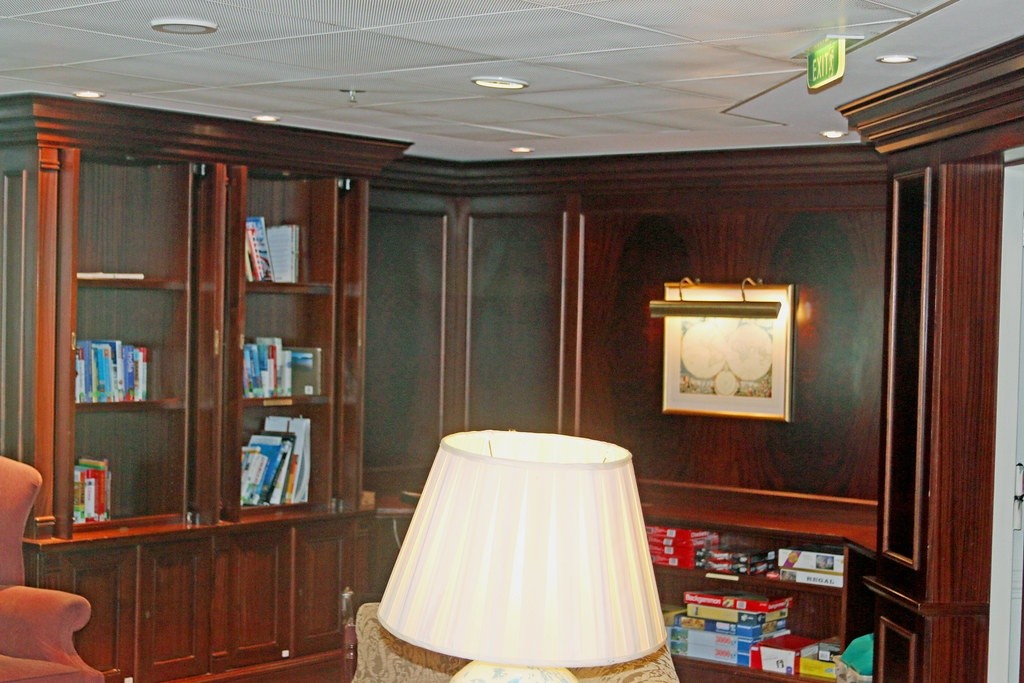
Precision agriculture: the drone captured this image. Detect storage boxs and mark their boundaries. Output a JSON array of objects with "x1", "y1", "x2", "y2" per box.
[{"x1": 647, "y1": 524, "x2": 844, "y2": 683}]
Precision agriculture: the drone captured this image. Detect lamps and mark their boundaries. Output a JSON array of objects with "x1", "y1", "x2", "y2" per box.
[
  {"x1": 647, "y1": 278, "x2": 784, "y2": 318},
  {"x1": 376, "y1": 430, "x2": 668, "y2": 683}
]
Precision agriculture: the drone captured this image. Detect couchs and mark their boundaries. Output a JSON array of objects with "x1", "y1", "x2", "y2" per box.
[{"x1": 0, "y1": 456, "x2": 105, "y2": 683}]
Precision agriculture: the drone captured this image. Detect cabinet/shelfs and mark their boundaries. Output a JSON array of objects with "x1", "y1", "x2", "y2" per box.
[
  {"x1": 0, "y1": 92, "x2": 416, "y2": 683},
  {"x1": 640, "y1": 501, "x2": 879, "y2": 683}
]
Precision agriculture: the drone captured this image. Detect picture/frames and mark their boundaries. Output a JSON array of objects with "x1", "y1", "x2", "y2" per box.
[{"x1": 664, "y1": 283, "x2": 798, "y2": 424}]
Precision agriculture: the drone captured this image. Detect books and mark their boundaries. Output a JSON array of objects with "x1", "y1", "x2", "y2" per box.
[
  {"x1": 243, "y1": 216, "x2": 301, "y2": 284},
  {"x1": 241, "y1": 337, "x2": 322, "y2": 398},
  {"x1": 240, "y1": 413, "x2": 311, "y2": 505},
  {"x1": 75, "y1": 457, "x2": 111, "y2": 523},
  {"x1": 74, "y1": 340, "x2": 148, "y2": 403}
]
